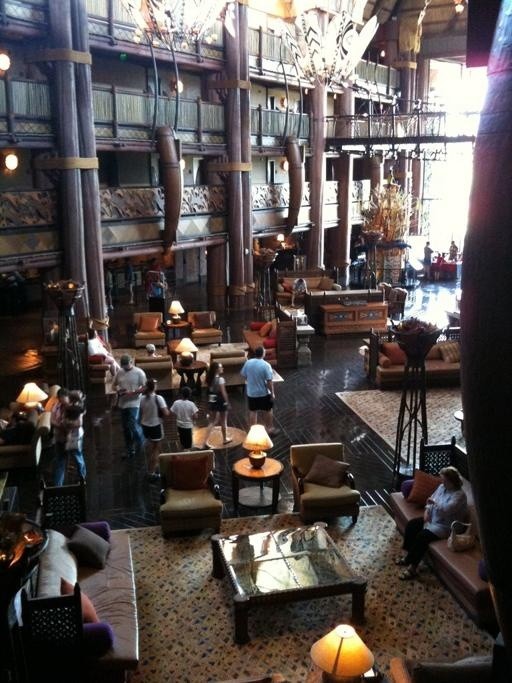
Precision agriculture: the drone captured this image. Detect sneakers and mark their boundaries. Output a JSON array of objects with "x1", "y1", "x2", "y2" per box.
[
  {"x1": 423, "y1": 276, "x2": 434, "y2": 280},
  {"x1": 204, "y1": 437, "x2": 232, "y2": 450},
  {"x1": 269, "y1": 428, "x2": 281, "y2": 435}
]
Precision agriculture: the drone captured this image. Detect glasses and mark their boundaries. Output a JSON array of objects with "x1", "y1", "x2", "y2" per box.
[{"x1": 218, "y1": 366, "x2": 224, "y2": 368}]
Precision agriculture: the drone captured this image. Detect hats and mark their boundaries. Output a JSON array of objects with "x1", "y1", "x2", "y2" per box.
[
  {"x1": 146, "y1": 343, "x2": 155, "y2": 352},
  {"x1": 121, "y1": 355, "x2": 132, "y2": 366}
]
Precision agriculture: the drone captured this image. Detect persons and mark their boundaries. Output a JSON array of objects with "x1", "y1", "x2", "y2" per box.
[
  {"x1": 87, "y1": 316, "x2": 120, "y2": 376},
  {"x1": 0, "y1": 386, "x2": 86, "y2": 513},
  {"x1": 111, "y1": 354, "x2": 148, "y2": 460},
  {"x1": 104, "y1": 256, "x2": 168, "y2": 327},
  {"x1": 202, "y1": 362, "x2": 233, "y2": 450},
  {"x1": 424, "y1": 240, "x2": 458, "y2": 281},
  {"x1": 137, "y1": 379, "x2": 170, "y2": 473},
  {"x1": 278, "y1": 278, "x2": 310, "y2": 308},
  {"x1": 146, "y1": 342, "x2": 161, "y2": 357},
  {"x1": 169, "y1": 386, "x2": 200, "y2": 452},
  {"x1": 240, "y1": 345, "x2": 281, "y2": 438},
  {"x1": 394, "y1": 466, "x2": 467, "y2": 582}
]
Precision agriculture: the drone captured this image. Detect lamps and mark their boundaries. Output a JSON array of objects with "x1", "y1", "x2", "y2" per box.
[
  {"x1": 272, "y1": 0, "x2": 380, "y2": 100},
  {"x1": 119, "y1": 0, "x2": 233, "y2": 54}
]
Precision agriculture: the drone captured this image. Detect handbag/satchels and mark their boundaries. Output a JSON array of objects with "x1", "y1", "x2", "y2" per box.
[
  {"x1": 207, "y1": 391, "x2": 228, "y2": 411},
  {"x1": 157, "y1": 408, "x2": 165, "y2": 418},
  {"x1": 447, "y1": 532, "x2": 474, "y2": 551}
]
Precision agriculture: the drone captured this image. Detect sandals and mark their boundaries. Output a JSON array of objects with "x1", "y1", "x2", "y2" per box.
[
  {"x1": 394, "y1": 557, "x2": 409, "y2": 566},
  {"x1": 398, "y1": 569, "x2": 416, "y2": 580}
]
